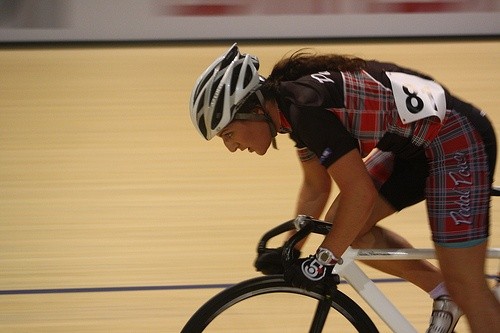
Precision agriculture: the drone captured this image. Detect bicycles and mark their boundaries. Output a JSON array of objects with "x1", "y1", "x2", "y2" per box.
[{"x1": 178, "y1": 186, "x2": 499, "y2": 333}]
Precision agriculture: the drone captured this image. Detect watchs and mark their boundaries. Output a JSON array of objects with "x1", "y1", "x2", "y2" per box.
[{"x1": 315, "y1": 246, "x2": 343, "y2": 267}]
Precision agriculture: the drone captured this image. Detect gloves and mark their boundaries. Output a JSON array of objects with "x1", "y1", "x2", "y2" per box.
[
  {"x1": 283, "y1": 254, "x2": 336, "y2": 293},
  {"x1": 254, "y1": 247, "x2": 300, "y2": 276}
]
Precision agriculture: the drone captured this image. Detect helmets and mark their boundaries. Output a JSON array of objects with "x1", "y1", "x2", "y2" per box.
[{"x1": 188, "y1": 42, "x2": 267, "y2": 141}]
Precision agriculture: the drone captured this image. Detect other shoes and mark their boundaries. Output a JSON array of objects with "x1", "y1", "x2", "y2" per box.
[{"x1": 425, "y1": 294, "x2": 465, "y2": 333}]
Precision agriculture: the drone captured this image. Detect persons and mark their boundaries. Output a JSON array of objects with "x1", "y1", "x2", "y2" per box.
[{"x1": 189, "y1": 42, "x2": 500, "y2": 333}]
